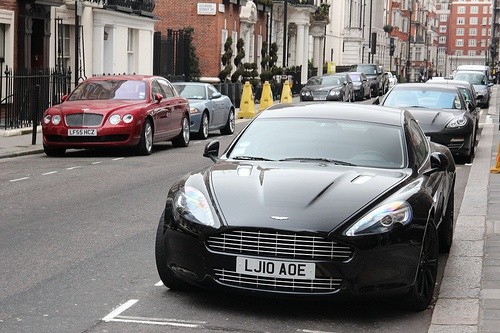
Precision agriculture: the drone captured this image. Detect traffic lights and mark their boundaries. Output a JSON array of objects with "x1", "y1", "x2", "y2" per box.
[
  {"x1": 490, "y1": 43, "x2": 496, "y2": 57},
  {"x1": 390, "y1": 38, "x2": 394, "y2": 56}
]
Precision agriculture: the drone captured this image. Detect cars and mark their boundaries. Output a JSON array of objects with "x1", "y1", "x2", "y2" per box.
[
  {"x1": 300, "y1": 63, "x2": 399, "y2": 103},
  {"x1": 381, "y1": 64, "x2": 497, "y2": 165},
  {"x1": 155, "y1": 101, "x2": 456, "y2": 311},
  {"x1": 42, "y1": 73, "x2": 235, "y2": 156}
]
